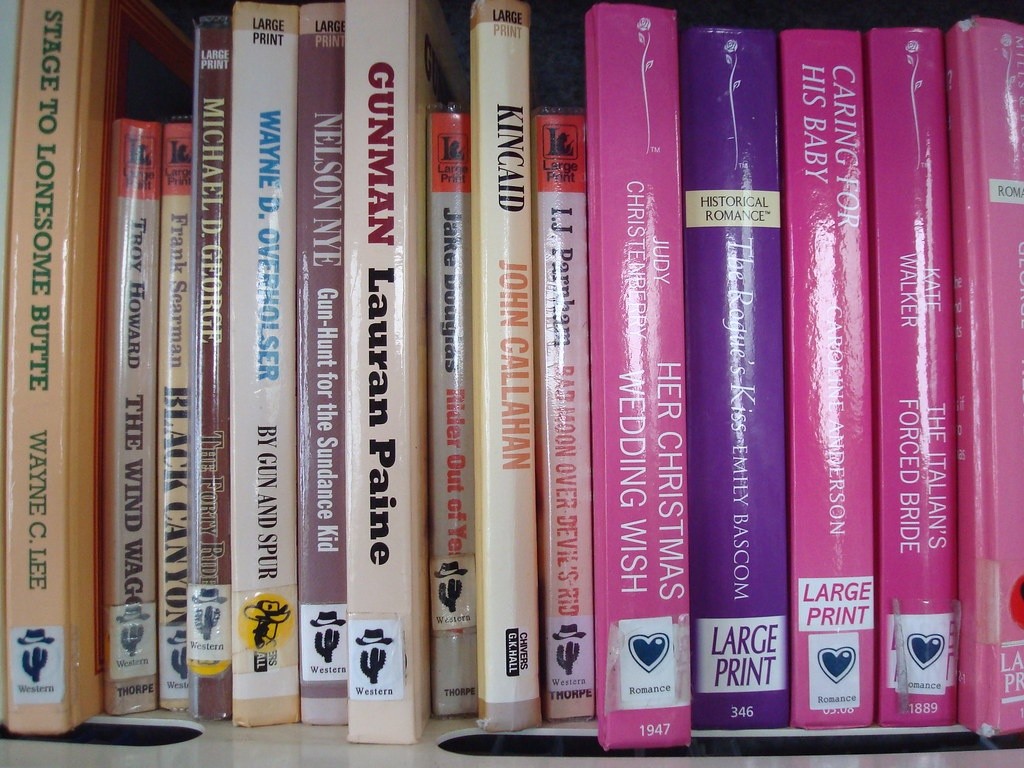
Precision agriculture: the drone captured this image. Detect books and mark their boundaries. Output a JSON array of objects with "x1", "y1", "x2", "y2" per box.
[{"x1": 0, "y1": 1, "x2": 1024, "y2": 750}]
